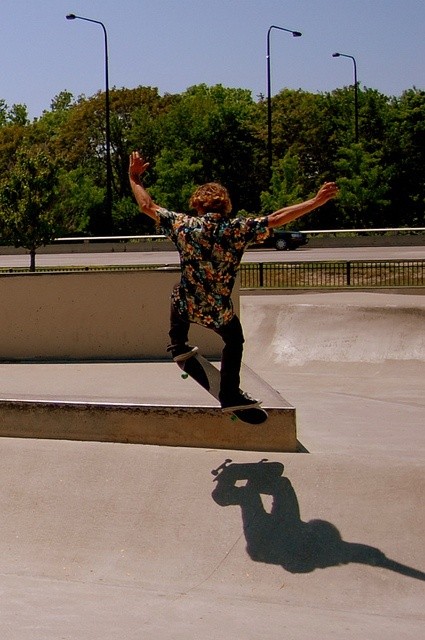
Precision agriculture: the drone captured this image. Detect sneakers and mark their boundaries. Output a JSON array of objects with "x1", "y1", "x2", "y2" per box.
[
  {"x1": 220, "y1": 390, "x2": 262, "y2": 413},
  {"x1": 167, "y1": 345, "x2": 199, "y2": 362}
]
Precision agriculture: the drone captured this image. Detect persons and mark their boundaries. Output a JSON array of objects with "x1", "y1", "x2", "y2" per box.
[{"x1": 127, "y1": 151, "x2": 340, "y2": 414}]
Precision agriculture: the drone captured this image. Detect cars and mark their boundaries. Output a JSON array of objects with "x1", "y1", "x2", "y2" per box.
[{"x1": 247, "y1": 228, "x2": 305, "y2": 250}]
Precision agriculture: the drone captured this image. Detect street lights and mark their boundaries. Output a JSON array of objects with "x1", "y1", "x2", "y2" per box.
[
  {"x1": 333, "y1": 53, "x2": 358, "y2": 173},
  {"x1": 66, "y1": 14, "x2": 112, "y2": 235},
  {"x1": 266, "y1": 25, "x2": 301, "y2": 214}
]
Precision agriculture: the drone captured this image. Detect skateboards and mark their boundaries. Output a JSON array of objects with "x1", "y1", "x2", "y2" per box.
[{"x1": 177, "y1": 353, "x2": 268, "y2": 424}]
